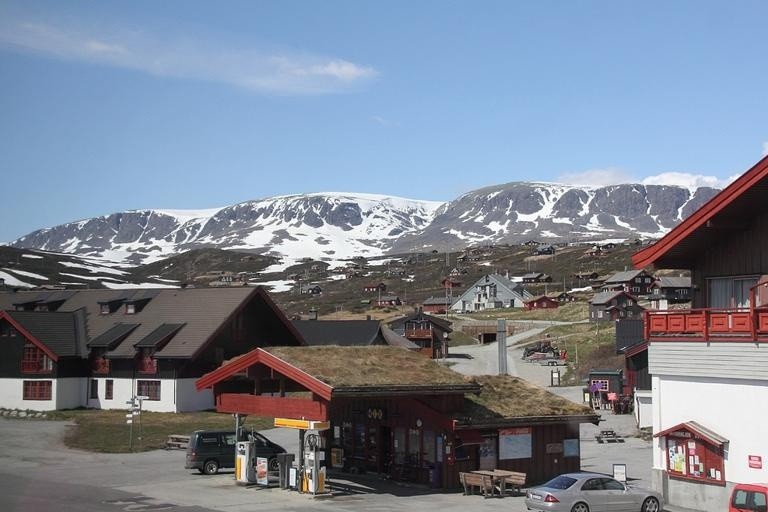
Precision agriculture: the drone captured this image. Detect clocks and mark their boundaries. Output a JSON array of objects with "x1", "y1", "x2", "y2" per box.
[{"x1": 416, "y1": 419, "x2": 423, "y2": 427}]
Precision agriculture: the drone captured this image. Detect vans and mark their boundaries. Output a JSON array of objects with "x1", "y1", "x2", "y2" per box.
[{"x1": 183, "y1": 429, "x2": 289, "y2": 475}]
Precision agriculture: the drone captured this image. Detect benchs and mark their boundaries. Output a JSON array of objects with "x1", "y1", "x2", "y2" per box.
[
  {"x1": 494, "y1": 469, "x2": 527, "y2": 497},
  {"x1": 167, "y1": 441, "x2": 191, "y2": 449},
  {"x1": 612, "y1": 430, "x2": 618, "y2": 436},
  {"x1": 595, "y1": 430, "x2": 600, "y2": 438},
  {"x1": 458, "y1": 471, "x2": 503, "y2": 499}
]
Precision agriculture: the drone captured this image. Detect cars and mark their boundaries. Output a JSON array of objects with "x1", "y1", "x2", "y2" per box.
[{"x1": 524, "y1": 472, "x2": 667, "y2": 511}]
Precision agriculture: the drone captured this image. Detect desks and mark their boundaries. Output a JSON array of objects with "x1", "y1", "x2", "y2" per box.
[
  {"x1": 470, "y1": 470, "x2": 511, "y2": 496},
  {"x1": 600, "y1": 426, "x2": 614, "y2": 437},
  {"x1": 167, "y1": 435, "x2": 190, "y2": 449}
]
